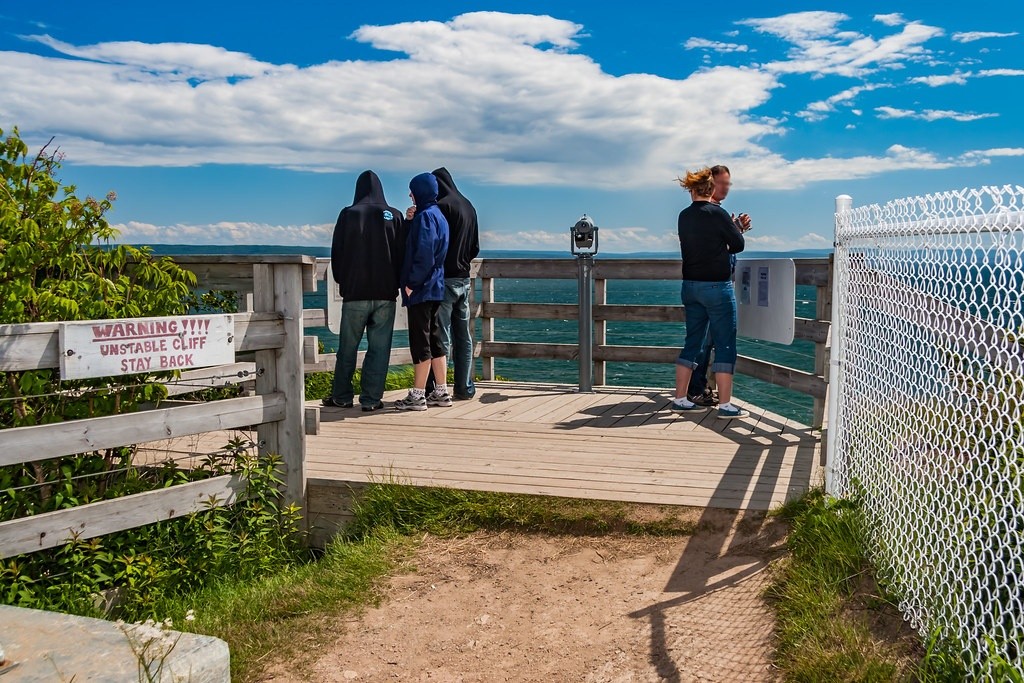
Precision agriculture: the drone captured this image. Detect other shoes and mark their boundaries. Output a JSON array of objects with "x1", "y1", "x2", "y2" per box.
[
  {"x1": 670, "y1": 399, "x2": 708, "y2": 413},
  {"x1": 717, "y1": 409, "x2": 750, "y2": 419}
]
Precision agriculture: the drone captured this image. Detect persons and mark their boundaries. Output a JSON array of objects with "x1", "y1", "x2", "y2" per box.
[
  {"x1": 393, "y1": 172, "x2": 454, "y2": 411},
  {"x1": 320, "y1": 169, "x2": 404, "y2": 413},
  {"x1": 425, "y1": 166, "x2": 483, "y2": 408},
  {"x1": 670, "y1": 165, "x2": 752, "y2": 421},
  {"x1": 688, "y1": 164, "x2": 753, "y2": 406}
]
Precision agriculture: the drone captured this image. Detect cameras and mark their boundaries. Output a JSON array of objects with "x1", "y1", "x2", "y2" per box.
[{"x1": 734, "y1": 212, "x2": 753, "y2": 235}]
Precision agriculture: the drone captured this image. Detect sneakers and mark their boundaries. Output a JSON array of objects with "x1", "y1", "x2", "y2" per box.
[
  {"x1": 322, "y1": 396, "x2": 353, "y2": 408},
  {"x1": 426, "y1": 390, "x2": 452, "y2": 407},
  {"x1": 394, "y1": 391, "x2": 428, "y2": 411},
  {"x1": 361, "y1": 400, "x2": 384, "y2": 411},
  {"x1": 687, "y1": 386, "x2": 719, "y2": 406}
]
